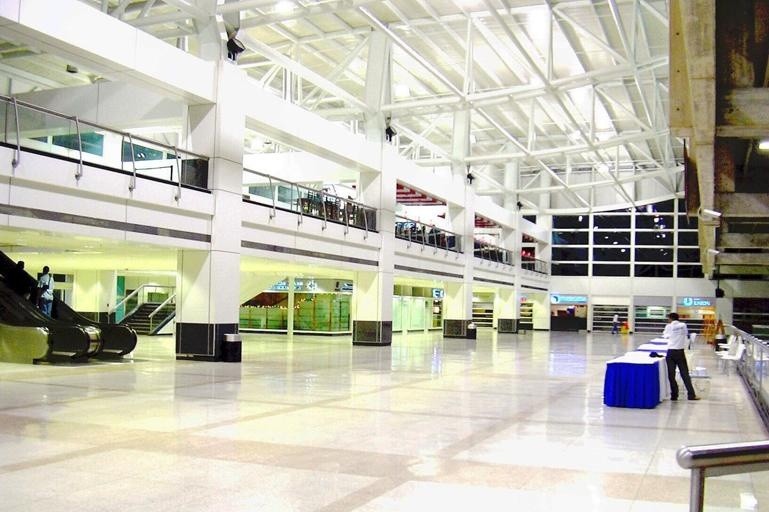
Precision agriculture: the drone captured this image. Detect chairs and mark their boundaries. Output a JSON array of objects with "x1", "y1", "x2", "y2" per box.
[
  {"x1": 687, "y1": 332, "x2": 697, "y2": 350},
  {"x1": 714, "y1": 332, "x2": 747, "y2": 375}
]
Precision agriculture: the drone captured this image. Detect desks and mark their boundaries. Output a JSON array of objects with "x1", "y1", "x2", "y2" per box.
[{"x1": 606, "y1": 335, "x2": 668, "y2": 410}]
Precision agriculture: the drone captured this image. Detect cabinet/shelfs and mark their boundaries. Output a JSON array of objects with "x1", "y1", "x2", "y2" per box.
[
  {"x1": 591, "y1": 305, "x2": 671, "y2": 334},
  {"x1": 519, "y1": 302, "x2": 533, "y2": 326},
  {"x1": 472, "y1": 300, "x2": 493, "y2": 326}
]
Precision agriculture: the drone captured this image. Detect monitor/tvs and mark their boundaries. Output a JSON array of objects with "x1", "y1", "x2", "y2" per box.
[
  {"x1": 433, "y1": 288, "x2": 443, "y2": 297},
  {"x1": 683, "y1": 139, "x2": 700, "y2": 224}
]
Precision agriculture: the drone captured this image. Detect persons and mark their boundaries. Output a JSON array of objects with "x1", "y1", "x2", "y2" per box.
[
  {"x1": 611, "y1": 312, "x2": 622, "y2": 334},
  {"x1": 11, "y1": 260, "x2": 31, "y2": 302},
  {"x1": 662, "y1": 312, "x2": 702, "y2": 400},
  {"x1": 34, "y1": 266, "x2": 55, "y2": 317},
  {"x1": 319, "y1": 185, "x2": 441, "y2": 245}
]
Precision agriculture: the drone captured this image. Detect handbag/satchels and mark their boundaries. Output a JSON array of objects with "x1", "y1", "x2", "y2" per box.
[{"x1": 42, "y1": 285, "x2": 49, "y2": 290}]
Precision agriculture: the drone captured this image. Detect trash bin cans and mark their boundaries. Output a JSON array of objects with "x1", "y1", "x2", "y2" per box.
[
  {"x1": 468, "y1": 324, "x2": 477, "y2": 339},
  {"x1": 714, "y1": 335, "x2": 727, "y2": 351},
  {"x1": 222, "y1": 334, "x2": 241, "y2": 362}
]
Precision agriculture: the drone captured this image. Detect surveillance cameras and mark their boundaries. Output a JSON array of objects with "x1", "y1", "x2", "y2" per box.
[
  {"x1": 702, "y1": 209, "x2": 723, "y2": 220},
  {"x1": 709, "y1": 248, "x2": 719, "y2": 256}
]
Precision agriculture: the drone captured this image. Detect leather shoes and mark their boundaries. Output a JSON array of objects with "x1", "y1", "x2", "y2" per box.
[
  {"x1": 693, "y1": 396, "x2": 702, "y2": 399},
  {"x1": 671, "y1": 397, "x2": 678, "y2": 400}
]
